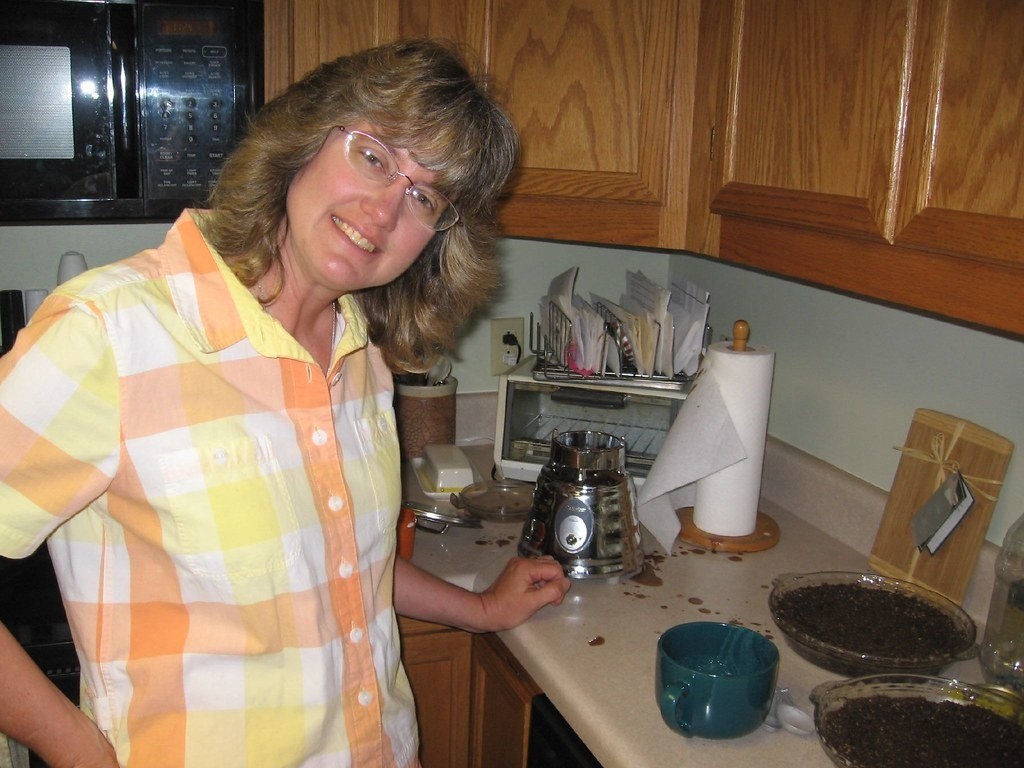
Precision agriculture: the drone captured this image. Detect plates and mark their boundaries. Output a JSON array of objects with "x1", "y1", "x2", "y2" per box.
[
  {"x1": 450, "y1": 478, "x2": 536, "y2": 523},
  {"x1": 808, "y1": 672, "x2": 1024, "y2": 768},
  {"x1": 766, "y1": 571, "x2": 990, "y2": 677},
  {"x1": 410, "y1": 456, "x2": 488, "y2": 502}
]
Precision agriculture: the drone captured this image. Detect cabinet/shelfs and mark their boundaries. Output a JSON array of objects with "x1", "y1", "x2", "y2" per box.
[
  {"x1": 264, "y1": 0, "x2": 702, "y2": 253},
  {"x1": 685, "y1": 0, "x2": 1024, "y2": 338}
]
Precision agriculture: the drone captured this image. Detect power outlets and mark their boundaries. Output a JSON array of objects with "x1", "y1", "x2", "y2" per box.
[{"x1": 491, "y1": 317, "x2": 524, "y2": 376}]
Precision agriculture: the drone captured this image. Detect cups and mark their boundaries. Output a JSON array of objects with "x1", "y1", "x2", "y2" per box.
[
  {"x1": 654, "y1": 621, "x2": 780, "y2": 740},
  {"x1": 397, "y1": 508, "x2": 417, "y2": 560}
]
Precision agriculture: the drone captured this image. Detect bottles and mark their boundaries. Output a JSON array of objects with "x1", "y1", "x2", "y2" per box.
[{"x1": 978, "y1": 508, "x2": 1024, "y2": 691}]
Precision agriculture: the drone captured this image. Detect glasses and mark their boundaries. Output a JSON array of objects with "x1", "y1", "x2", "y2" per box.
[{"x1": 338, "y1": 125, "x2": 460, "y2": 232}]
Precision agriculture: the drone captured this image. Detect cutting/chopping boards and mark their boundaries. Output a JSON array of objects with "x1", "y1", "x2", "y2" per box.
[{"x1": 869, "y1": 407, "x2": 1014, "y2": 606}]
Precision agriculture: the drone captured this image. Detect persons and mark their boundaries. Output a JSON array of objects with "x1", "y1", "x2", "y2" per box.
[{"x1": 0, "y1": 36, "x2": 570, "y2": 768}]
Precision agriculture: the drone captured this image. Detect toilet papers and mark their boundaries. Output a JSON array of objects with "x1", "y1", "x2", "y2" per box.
[{"x1": 634, "y1": 341, "x2": 777, "y2": 556}]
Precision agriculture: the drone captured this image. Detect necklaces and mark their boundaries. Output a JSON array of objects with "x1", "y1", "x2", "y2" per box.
[{"x1": 257, "y1": 283, "x2": 335, "y2": 379}]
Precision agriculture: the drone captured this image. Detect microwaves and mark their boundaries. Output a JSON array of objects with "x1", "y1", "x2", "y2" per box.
[{"x1": 0, "y1": 0, "x2": 257, "y2": 221}]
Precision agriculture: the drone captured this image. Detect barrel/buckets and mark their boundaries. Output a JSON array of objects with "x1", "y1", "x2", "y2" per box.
[{"x1": 393, "y1": 375, "x2": 458, "y2": 462}]
